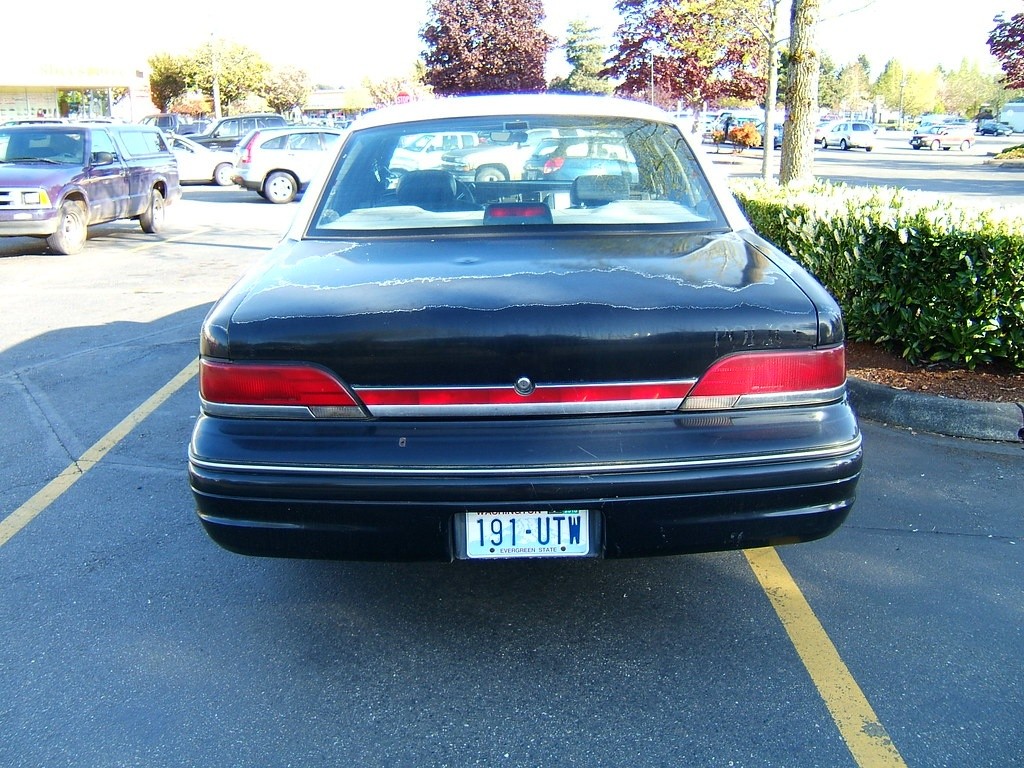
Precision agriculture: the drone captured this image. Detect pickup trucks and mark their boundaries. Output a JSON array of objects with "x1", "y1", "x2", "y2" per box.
[{"x1": 137, "y1": 115, "x2": 207, "y2": 136}]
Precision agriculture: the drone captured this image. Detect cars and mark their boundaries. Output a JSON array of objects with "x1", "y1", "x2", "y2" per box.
[
  {"x1": 705, "y1": 114, "x2": 784, "y2": 151},
  {"x1": 231, "y1": 125, "x2": 346, "y2": 205},
  {"x1": 981, "y1": 123, "x2": 1014, "y2": 137},
  {"x1": 437, "y1": 128, "x2": 597, "y2": 182},
  {"x1": 815, "y1": 114, "x2": 880, "y2": 145},
  {"x1": 822, "y1": 122, "x2": 873, "y2": 153},
  {"x1": 184, "y1": 91, "x2": 867, "y2": 574},
  {"x1": 916, "y1": 115, "x2": 979, "y2": 133},
  {"x1": 518, "y1": 136, "x2": 642, "y2": 186},
  {"x1": 164, "y1": 135, "x2": 238, "y2": 186},
  {"x1": 390, "y1": 133, "x2": 479, "y2": 187}
]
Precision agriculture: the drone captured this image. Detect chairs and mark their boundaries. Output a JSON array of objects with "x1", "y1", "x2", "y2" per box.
[
  {"x1": 395, "y1": 169, "x2": 457, "y2": 210},
  {"x1": 567, "y1": 175, "x2": 629, "y2": 209}
]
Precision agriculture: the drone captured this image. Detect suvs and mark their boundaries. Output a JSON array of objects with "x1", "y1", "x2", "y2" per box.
[
  {"x1": 0, "y1": 124, "x2": 180, "y2": 257},
  {"x1": 183, "y1": 113, "x2": 289, "y2": 155},
  {"x1": 909, "y1": 125, "x2": 976, "y2": 153}
]
